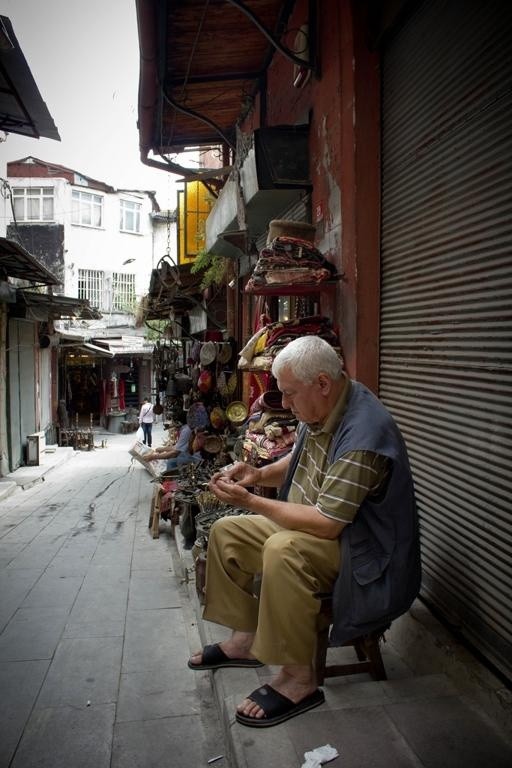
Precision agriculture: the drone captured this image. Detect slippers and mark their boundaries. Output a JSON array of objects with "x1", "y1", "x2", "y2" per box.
[
  {"x1": 235, "y1": 684, "x2": 325, "y2": 729},
  {"x1": 187, "y1": 644, "x2": 265, "y2": 670}
]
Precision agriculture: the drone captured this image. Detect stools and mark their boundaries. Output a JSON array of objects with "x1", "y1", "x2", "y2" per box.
[{"x1": 310, "y1": 612, "x2": 389, "y2": 689}]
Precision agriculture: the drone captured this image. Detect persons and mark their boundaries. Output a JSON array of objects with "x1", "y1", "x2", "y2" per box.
[
  {"x1": 139, "y1": 397, "x2": 154, "y2": 447},
  {"x1": 187, "y1": 336, "x2": 419, "y2": 726},
  {"x1": 141, "y1": 411, "x2": 192, "y2": 478}
]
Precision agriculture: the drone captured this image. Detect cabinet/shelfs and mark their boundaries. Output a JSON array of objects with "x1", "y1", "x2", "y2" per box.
[{"x1": 237, "y1": 280, "x2": 339, "y2": 499}]
